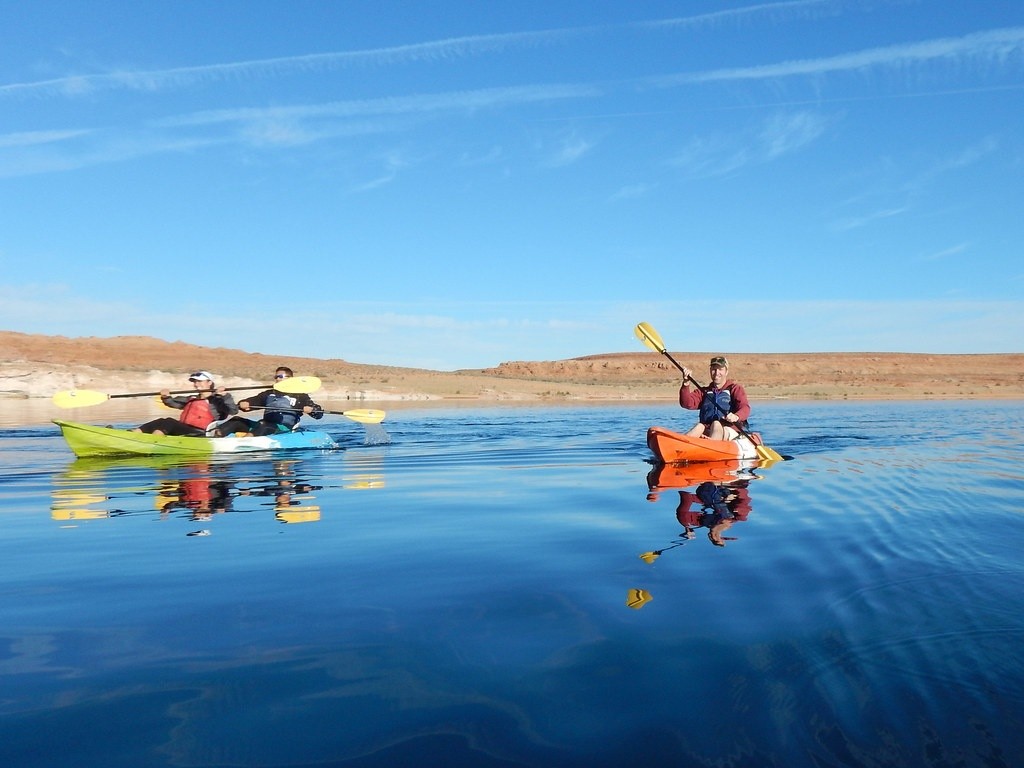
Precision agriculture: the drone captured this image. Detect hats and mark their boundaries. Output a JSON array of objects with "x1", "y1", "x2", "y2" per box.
[
  {"x1": 709, "y1": 357, "x2": 729, "y2": 369},
  {"x1": 188, "y1": 371, "x2": 214, "y2": 381}
]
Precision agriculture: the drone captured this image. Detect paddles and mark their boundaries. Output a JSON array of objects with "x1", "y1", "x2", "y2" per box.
[
  {"x1": 640, "y1": 522, "x2": 698, "y2": 565},
  {"x1": 54, "y1": 503, "x2": 331, "y2": 526},
  {"x1": 247, "y1": 405, "x2": 386, "y2": 426},
  {"x1": 51, "y1": 375, "x2": 323, "y2": 410},
  {"x1": 634, "y1": 321, "x2": 784, "y2": 461}
]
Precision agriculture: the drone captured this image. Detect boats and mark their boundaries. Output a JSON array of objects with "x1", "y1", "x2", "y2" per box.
[
  {"x1": 647, "y1": 459, "x2": 760, "y2": 501},
  {"x1": 57, "y1": 450, "x2": 320, "y2": 482},
  {"x1": 646, "y1": 427, "x2": 762, "y2": 463},
  {"x1": 52, "y1": 420, "x2": 340, "y2": 456}
]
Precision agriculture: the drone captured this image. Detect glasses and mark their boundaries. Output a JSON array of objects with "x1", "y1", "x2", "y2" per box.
[
  {"x1": 274, "y1": 374, "x2": 290, "y2": 379},
  {"x1": 711, "y1": 358, "x2": 726, "y2": 364},
  {"x1": 191, "y1": 372, "x2": 209, "y2": 378}
]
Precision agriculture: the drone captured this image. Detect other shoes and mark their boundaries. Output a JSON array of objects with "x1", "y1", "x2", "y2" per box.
[{"x1": 106, "y1": 425, "x2": 113, "y2": 429}]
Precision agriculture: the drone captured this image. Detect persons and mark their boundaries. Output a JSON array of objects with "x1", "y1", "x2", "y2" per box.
[
  {"x1": 678, "y1": 356, "x2": 751, "y2": 441},
  {"x1": 106, "y1": 372, "x2": 239, "y2": 435},
  {"x1": 207, "y1": 367, "x2": 323, "y2": 438}
]
